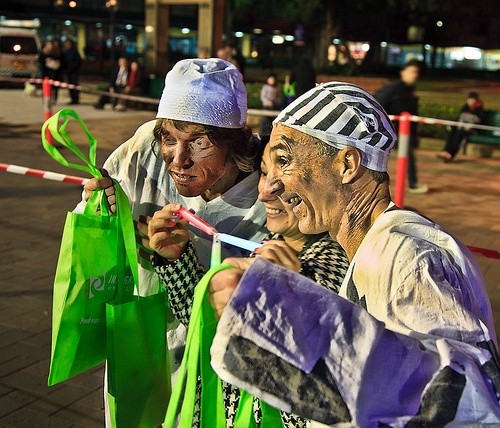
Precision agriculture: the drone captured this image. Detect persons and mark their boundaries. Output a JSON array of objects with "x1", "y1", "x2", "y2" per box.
[
  {"x1": 436, "y1": 90, "x2": 484, "y2": 163},
  {"x1": 286, "y1": 40, "x2": 317, "y2": 100},
  {"x1": 147, "y1": 118, "x2": 350, "y2": 428},
  {"x1": 91, "y1": 56, "x2": 130, "y2": 110},
  {"x1": 33, "y1": 39, "x2": 84, "y2": 105},
  {"x1": 217, "y1": 43, "x2": 246, "y2": 84},
  {"x1": 373, "y1": 59, "x2": 430, "y2": 194},
  {"x1": 260, "y1": 74, "x2": 281, "y2": 135},
  {"x1": 69, "y1": 58, "x2": 268, "y2": 428},
  {"x1": 208, "y1": 81, "x2": 499, "y2": 428},
  {"x1": 113, "y1": 60, "x2": 150, "y2": 111}
]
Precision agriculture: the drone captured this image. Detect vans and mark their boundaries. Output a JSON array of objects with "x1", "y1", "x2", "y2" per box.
[{"x1": 1, "y1": 28, "x2": 44, "y2": 86}]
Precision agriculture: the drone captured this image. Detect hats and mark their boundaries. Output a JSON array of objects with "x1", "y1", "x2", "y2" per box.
[
  {"x1": 272, "y1": 81, "x2": 398, "y2": 171},
  {"x1": 155, "y1": 58, "x2": 247, "y2": 128}
]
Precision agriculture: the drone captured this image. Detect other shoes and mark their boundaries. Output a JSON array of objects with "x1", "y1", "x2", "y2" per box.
[
  {"x1": 436, "y1": 151, "x2": 451, "y2": 161},
  {"x1": 411, "y1": 188, "x2": 429, "y2": 193}
]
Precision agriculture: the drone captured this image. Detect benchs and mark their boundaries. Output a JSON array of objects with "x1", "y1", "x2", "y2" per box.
[{"x1": 445, "y1": 107, "x2": 500, "y2": 158}]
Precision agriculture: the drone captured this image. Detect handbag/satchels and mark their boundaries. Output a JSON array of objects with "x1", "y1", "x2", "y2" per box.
[
  {"x1": 42, "y1": 108, "x2": 134, "y2": 387},
  {"x1": 99, "y1": 178, "x2": 172, "y2": 428}
]
